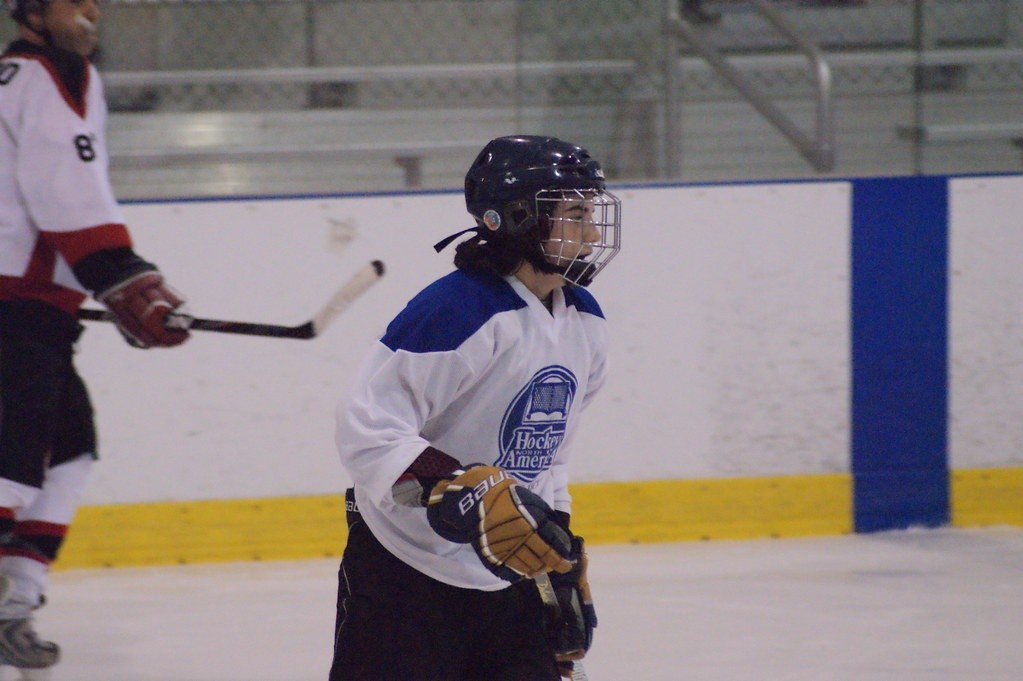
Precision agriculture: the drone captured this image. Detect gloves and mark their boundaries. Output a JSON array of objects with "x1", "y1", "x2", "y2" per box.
[
  {"x1": 94, "y1": 259, "x2": 191, "y2": 350},
  {"x1": 539, "y1": 535, "x2": 598, "y2": 678},
  {"x1": 428, "y1": 462, "x2": 579, "y2": 580}
]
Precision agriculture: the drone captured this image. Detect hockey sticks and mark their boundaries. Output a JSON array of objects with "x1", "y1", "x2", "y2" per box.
[
  {"x1": 534, "y1": 573, "x2": 588, "y2": 681},
  {"x1": 75, "y1": 259, "x2": 386, "y2": 342}
]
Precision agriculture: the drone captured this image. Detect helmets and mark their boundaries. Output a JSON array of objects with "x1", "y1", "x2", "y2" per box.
[{"x1": 465, "y1": 134, "x2": 622, "y2": 287}]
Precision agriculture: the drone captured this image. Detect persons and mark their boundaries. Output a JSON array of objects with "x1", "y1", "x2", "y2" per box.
[
  {"x1": 327, "y1": 133, "x2": 622, "y2": 681},
  {"x1": 0, "y1": 0, "x2": 191, "y2": 668}
]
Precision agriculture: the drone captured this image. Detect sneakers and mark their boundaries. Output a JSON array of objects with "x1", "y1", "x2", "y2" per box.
[{"x1": 0, "y1": 619, "x2": 61, "y2": 680}]
